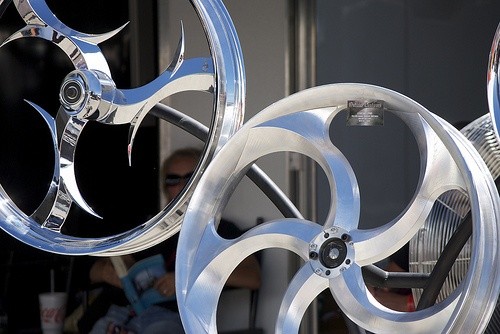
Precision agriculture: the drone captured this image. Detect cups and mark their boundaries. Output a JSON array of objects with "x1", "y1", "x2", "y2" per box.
[{"x1": 39, "y1": 293, "x2": 67, "y2": 333}]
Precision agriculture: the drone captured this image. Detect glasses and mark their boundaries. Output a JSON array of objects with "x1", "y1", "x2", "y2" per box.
[{"x1": 163, "y1": 172, "x2": 193, "y2": 186}]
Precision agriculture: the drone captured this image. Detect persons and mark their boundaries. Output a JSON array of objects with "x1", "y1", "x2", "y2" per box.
[
  {"x1": 367, "y1": 120, "x2": 500, "y2": 314},
  {"x1": 76, "y1": 143, "x2": 263, "y2": 334}
]
